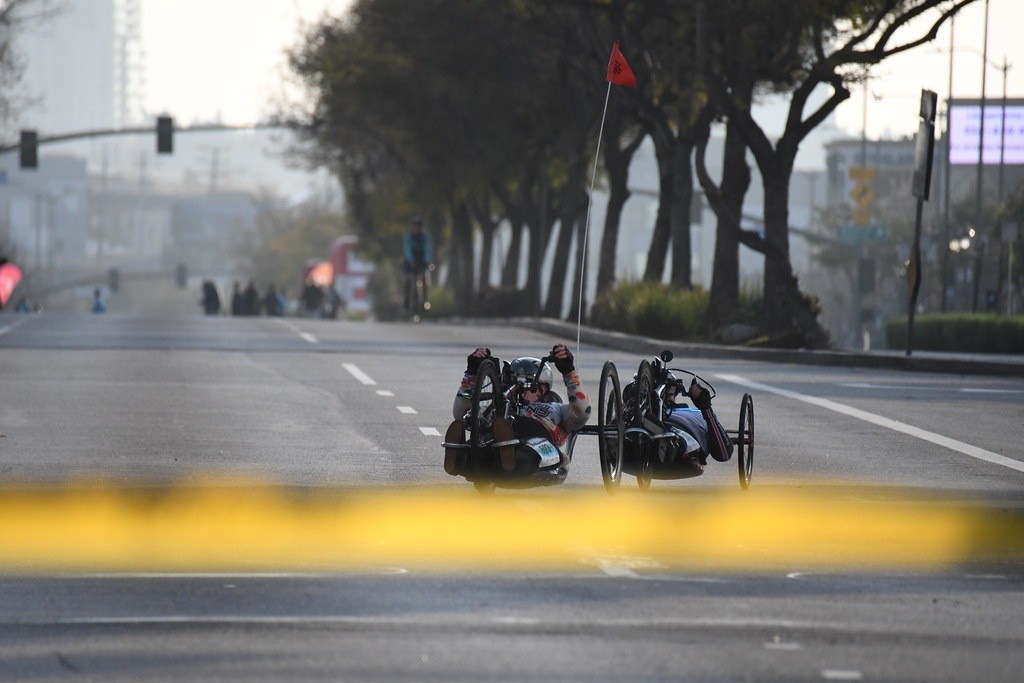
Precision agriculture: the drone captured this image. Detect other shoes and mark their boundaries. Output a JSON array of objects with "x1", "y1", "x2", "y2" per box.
[
  {"x1": 443, "y1": 420, "x2": 466, "y2": 476},
  {"x1": 643, "y1": 413, "x2": 676, "y2": 466},
  {"x1": 492, "y1": 417, "x2": 516, "y2": 472}
]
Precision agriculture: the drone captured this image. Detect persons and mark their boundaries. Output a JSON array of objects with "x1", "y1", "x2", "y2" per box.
[
  {"x1": 606, "y1": 366, "x2": 734, "y2": 480},
  {"x1": 198, "y1": 279, "x2": 288, "y2": 317},
  {"x1": 15, "y1": 296, "x2": 29, "y2": 313},
  {"x1": 400, "y1": 216, "x2": 433, "y2": 317},
  {"x1": 299, "y1": 286, "x2": 323, "y2": 317},
  {"x1": 91, "y1": 289, "x2": 105, "y2": 314},
  {"x1": 452, "y1": 342, "x2": 592, "y2": 483}
]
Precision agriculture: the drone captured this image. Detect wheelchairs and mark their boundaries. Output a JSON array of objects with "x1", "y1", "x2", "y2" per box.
[{"x1": 443, "y1": 345, "x2": 754, "y2": 492}]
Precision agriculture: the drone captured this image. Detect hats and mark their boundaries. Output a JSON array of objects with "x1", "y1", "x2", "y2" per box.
[{"x1": 411, "y1": 216, "x2": 424, "y2": 226}]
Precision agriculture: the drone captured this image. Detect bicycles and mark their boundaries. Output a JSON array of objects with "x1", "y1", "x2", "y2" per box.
[{"x1": 399, "y1": 262, "x2": 435, "y2": 324}]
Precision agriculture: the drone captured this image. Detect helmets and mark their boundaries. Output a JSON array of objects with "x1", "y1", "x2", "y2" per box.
[{"x1": 510, "y1": 357, "x2": 553, "y2": 392}]
